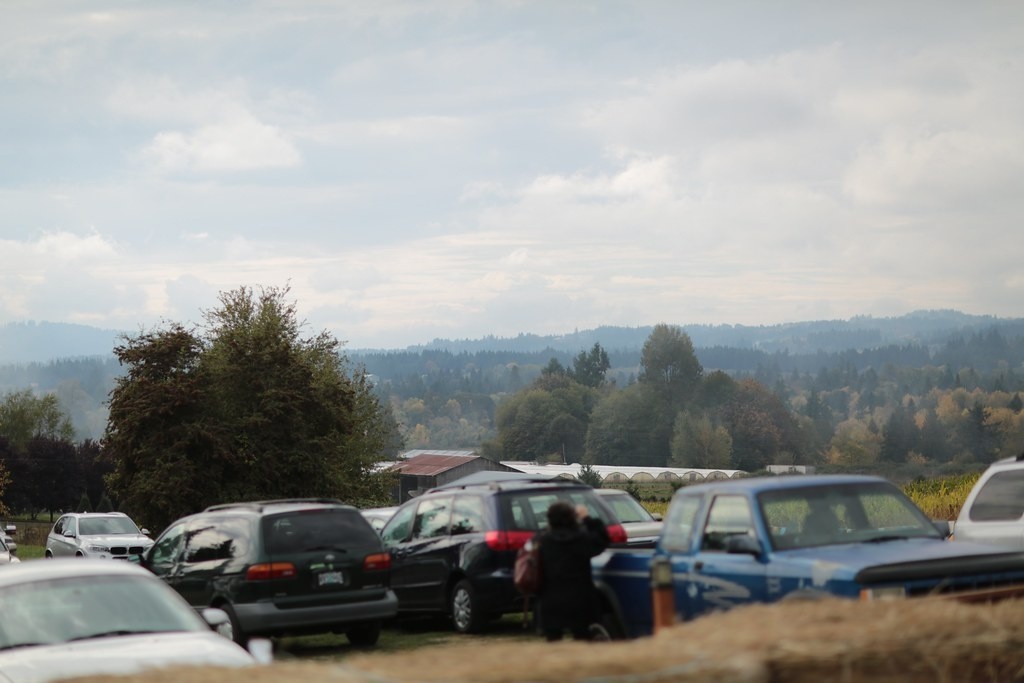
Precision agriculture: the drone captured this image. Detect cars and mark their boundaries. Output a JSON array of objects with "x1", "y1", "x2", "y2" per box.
[
  {"x1": 529, "y1": 487, "x2": 665, "y2": 549},
  {"x1": 0, "y1": 557, "x2": 262, "y2": 683},
  {"x1": 359, "y1": 505, "x2": 399, "y2": 533},
  {"x1": 0, "y1": 525, "x2": 18, "y2": 553},
  {"x1": 0, "y1": 536, "x2": 20, "y2": 565}
]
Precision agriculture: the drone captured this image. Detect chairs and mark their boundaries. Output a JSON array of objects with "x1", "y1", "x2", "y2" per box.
[{"x1": 797, "y1": 507, "x2": 851, "y2": 549}]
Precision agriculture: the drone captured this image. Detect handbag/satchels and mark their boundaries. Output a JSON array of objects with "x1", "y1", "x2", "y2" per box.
[{"x1": 513, "y1": 537, "x2": 543, "y2": 603}]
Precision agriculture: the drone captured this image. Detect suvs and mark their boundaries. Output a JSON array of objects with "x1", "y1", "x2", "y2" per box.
[
  {"x1": 378, "y1": 473, "x2": 631, "y2": 637},
  {"x1": 947, "y1": 456, "x2": 1023, "y2": 554},
  {"x1": 125, "y1": 497, "x2": 400, "y2": 653},
  {"x1": 45, "y1": 512, "x2": 154, "y2": 560}
]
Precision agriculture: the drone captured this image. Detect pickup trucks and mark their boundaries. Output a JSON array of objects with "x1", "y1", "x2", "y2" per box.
[{"x1": 589, "y1": 472, "x2": 1023, "y2": 639}]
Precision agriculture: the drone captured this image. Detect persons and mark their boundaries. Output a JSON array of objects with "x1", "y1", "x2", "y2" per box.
[{"x1": 523, "y1": 503, "x2": 611, "y2": 641}]
